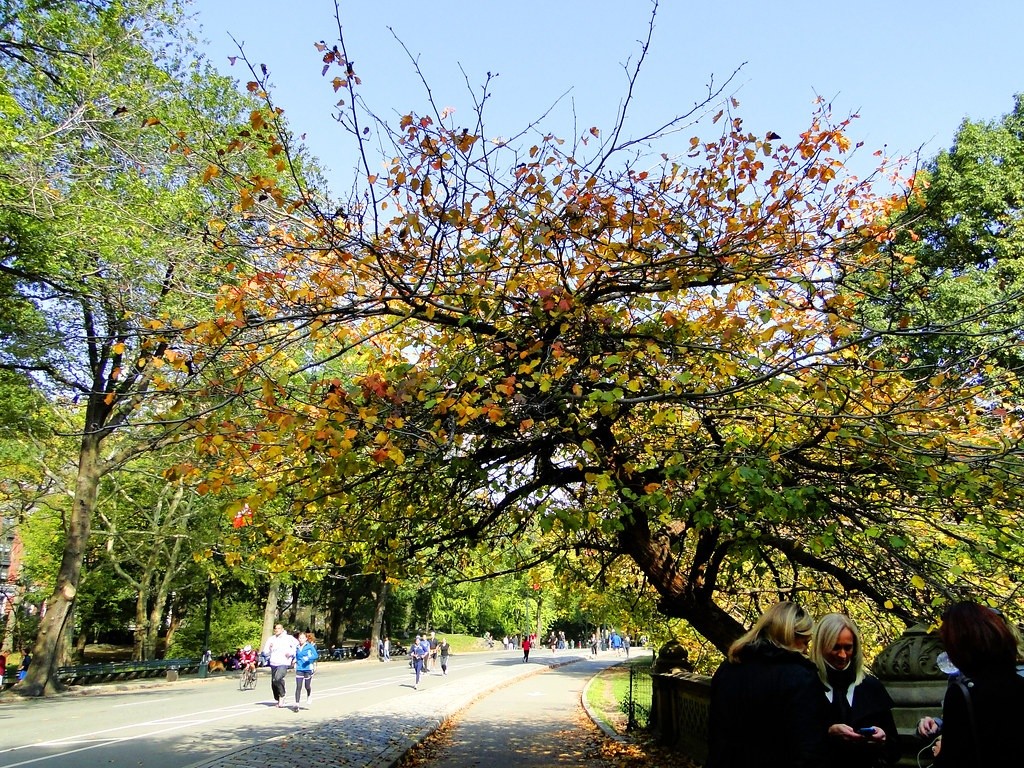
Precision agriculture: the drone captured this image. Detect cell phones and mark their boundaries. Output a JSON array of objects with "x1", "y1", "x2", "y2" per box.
[{"x1": 856, "y1": 727, "x2": 876, "y2": 736}]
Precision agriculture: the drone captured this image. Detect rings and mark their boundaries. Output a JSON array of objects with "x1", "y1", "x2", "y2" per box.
[{"x1": 932, "y1": 746, "x2": 936, "y2": 751}]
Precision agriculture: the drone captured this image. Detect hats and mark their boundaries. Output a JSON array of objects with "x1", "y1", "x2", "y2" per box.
[{"x1": 22, "y1": 645, "x2": 31, "y2": 655}]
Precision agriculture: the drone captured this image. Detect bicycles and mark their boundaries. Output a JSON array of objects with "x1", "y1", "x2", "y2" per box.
[{"x1": 238, "y1": 662, "x2": 258, "y2": 691}]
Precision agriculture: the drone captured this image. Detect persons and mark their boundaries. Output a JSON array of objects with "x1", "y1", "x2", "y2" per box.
[
  {"x1": 380, "y1": 637, "x2": 408, "y2": 664},
  {"x1": 588, "y1": 631, "x2": 646, "y2": 658},
  {"x1": 408, "y1": 632, "x2": 452, "y2": 690},
  {"x1": 291, "y1": 631, "x2": 319, "y2": 713},
  {"x1": 522, "y1": 636, "x2": 531, "y2": 663},
  {"x1": 707, "y1": 599, "x2": 1024, "y2": 768},
  {"x1": 351, "y1": 637, "x2": 371, "y2": 659},
  {"x1": 239, "y1": 645, "x2": 257, "y2": 682},
  {"x1": 548, "y1": 631, "x2": 575, "y2": 653},
  {"x1": 17, "y1": 649, "x2": 31, "y2": 682},
  {"x1": 330, "y1": 644, "x2": 345, "y2": 661},
  {"x1": 306, "y1": 632, "x2": 318, "y2": 675},
  {"x1": 0, "y1": 648, "x2": 12, "y2": 692},
  {"x1": 484, "y1": 630, "x2": 518, "y2": 650},
  {"x1": 203, "y1": 649, "x2": 267, "y2": 674},
  {"x1": 263, "y1": 624, "x2": 301, "y2": 708}
]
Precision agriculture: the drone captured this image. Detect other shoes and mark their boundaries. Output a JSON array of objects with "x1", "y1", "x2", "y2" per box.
[
  {"x1": 292, "y1": 702, "x2": 299, "y2": 712},
  {"x1": 522, "y1": 657, "x2": 525, "y2": 663},
  {"x1": 303, "y1": 702, "x2": 312, "y2": 709},
  {"x1": 414, "y1": 684, "x2": 418, "y2": 690},
  {"x1": 252, "y1": 675, "x2": 256, "y2": 680},
  {"x1": 279, "y1": 692, "x2": 286, "y2": 708}
]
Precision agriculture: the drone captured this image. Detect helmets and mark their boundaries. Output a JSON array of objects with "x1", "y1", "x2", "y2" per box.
[{"x1": 243, "y1": 645, "x2": 251, "y2": 651}]
